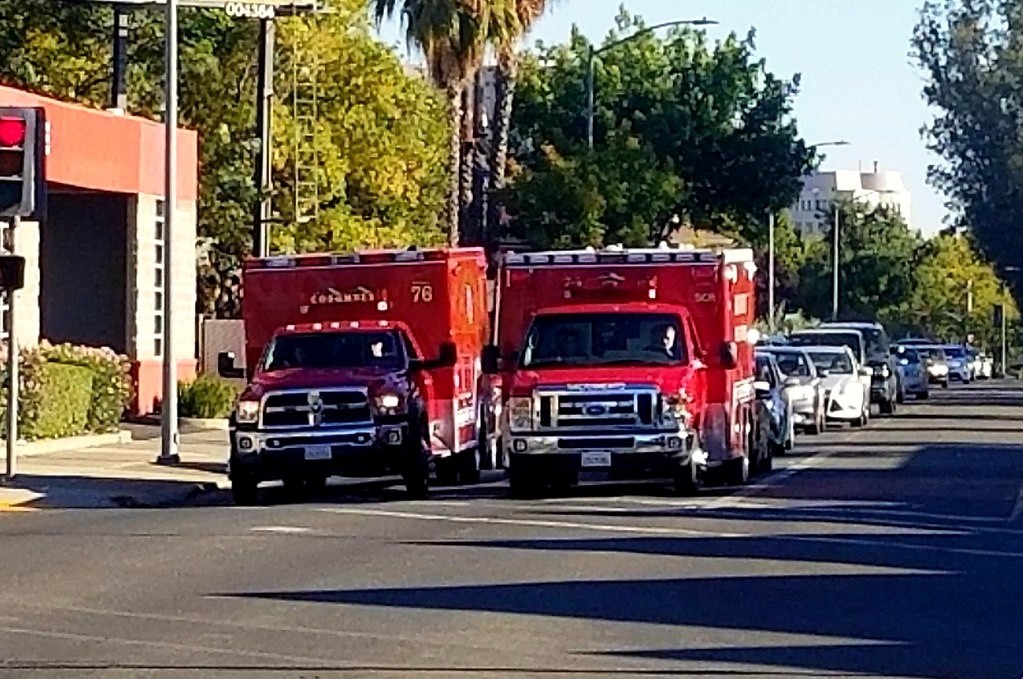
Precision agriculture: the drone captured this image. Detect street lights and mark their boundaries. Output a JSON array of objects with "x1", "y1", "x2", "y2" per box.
[
  {"x1": 769, "y1": 141, "x2": 850, "y2": 326},
  {"x1": 587, "y1": 21, "x2": 718, "y2": 155},
  {"x1": 832, "y1": 189, "x2": 895, "y2": 326}
]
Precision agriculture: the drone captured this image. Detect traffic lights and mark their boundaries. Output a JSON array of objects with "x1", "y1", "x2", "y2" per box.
[{"x1": 0, "y1": 105, "x2": 34, "y2": 220}]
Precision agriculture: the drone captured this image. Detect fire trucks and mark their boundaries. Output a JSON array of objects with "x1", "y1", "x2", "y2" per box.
[
  {"x1": 480, "y1": 245, "x2": 758, "y2": 499},
  {"x1": 226, "y1": 247, "x2": 489, "y2": 506}
]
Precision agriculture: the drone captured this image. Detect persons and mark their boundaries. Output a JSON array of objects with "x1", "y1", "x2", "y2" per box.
[
  {"x1": 284, "y1": 345, "x2": 307, "y2": 365},
  {"x1": 363, "y1": 332, "x2": 399, "y2": 370},
  {"x1": 547, "y1": 326, "x2": 588, "y2": 358},
  {"x1": 645, "y1": 324, "x2": 678, "y2": 362}
]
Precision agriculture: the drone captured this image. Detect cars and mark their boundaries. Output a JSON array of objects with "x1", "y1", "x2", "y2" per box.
[{"x1": 750, "y1": 323, "x2": 993, "y2": 480}]
